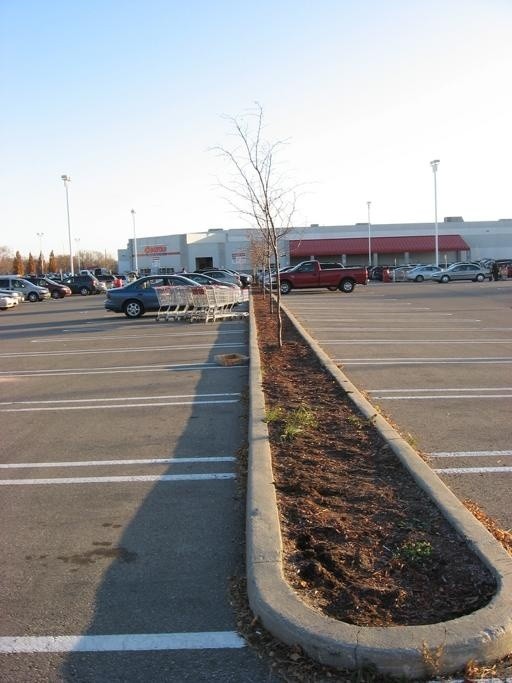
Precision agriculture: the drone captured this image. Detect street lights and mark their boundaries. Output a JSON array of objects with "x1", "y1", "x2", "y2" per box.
[
  {"x1": 130, "y1": 209, "x2": 138, "y2": 275},
  {"x1": 429, "y1": 160, "x2": 442, "y2": 267},
  {"x1": 60, "y1": 174, "x2": 76, "y2": 279},
  {"x1": 365, "y1": 201, "x2": 373, "y2": 267}
]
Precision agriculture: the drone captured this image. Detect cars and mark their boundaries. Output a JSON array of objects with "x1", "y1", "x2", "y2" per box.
[
  {"x1": 368, "y1": 263, "x2": 443, "y2": 282},
  {"x1": 0, "y1": 274, "x2": 73, "y2": 311},
  {"x1": 46, "y1": 268, "x2": 128, "y2": 296},
  {"x1": 103, "y1": 267, "x2": 254, "y2": 319},
  {"x1": 258, "y1": 261, "x2": 346, "y2": 289},
  {"x1": 431, "y1": 259, "x2": 498, "y2": 283}
]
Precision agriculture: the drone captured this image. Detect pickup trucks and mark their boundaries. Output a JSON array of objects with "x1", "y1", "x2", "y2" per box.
[{"x1": 276, "y1": 259, "x2": 369, "y2": 295}]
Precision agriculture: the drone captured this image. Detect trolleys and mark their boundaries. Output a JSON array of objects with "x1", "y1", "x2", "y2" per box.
[{"x1": 151, "y1": 285, "x2": 250, "y2": 324}]
